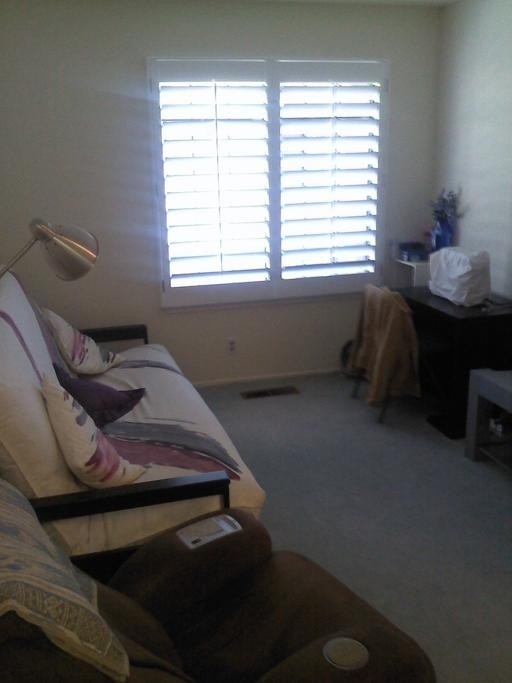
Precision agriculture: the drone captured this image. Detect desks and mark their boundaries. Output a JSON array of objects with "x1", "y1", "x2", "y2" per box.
[{"x1": 388, "y1": 284, "x2": 511, "y2": 435}]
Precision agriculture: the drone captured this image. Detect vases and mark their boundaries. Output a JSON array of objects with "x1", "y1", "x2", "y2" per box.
[{"x1": 430, "y1": 223, "x2": 455, "y2": 250}]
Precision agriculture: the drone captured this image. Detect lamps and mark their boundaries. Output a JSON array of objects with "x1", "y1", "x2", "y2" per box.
[{"x1": 1, "y1": 218, "x2": 99, "y2": 281}]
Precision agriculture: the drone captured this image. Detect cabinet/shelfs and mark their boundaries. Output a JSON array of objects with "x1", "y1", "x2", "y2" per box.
[{"x1": 468, "y1": 370, "x2": 511, "y2": 474}]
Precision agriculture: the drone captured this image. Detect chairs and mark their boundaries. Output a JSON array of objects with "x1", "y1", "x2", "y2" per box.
[
  {"x1": 350, "y1": 284, "x2": 440, "y2": 424},
  {"x1": 1, "y1": 474, "x2": 436, "y2": 683}
]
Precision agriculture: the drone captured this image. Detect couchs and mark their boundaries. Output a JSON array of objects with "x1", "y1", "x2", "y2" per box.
[{"x1": 1, "y1": 271, "x2": 267, "y2": 581}]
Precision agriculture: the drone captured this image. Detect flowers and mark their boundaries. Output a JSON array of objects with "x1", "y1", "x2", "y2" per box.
[{"x1": 426, "y1": 186, "x2": 460, "y2": 227}]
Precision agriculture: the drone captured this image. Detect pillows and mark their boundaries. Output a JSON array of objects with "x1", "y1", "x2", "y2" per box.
[{"x1": 34, "y1": 307, "x2": 148, "y2": 486}]
